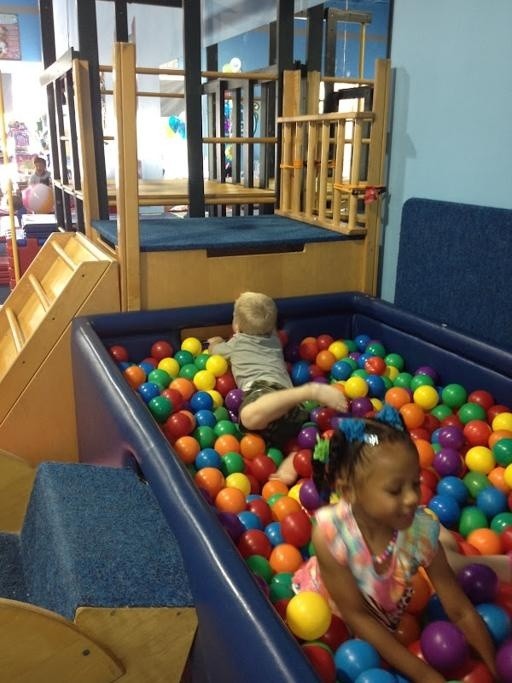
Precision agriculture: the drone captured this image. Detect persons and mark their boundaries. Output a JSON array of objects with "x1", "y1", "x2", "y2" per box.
[
  {"x1": 17, "y1": 157, "x2": 52, "y2": 228},
  {"x1": 313, "y1": 419, "x2": 501, "y2": 682},
  {"x1": 208, "y1": 290, "x2": 347, "y2": 483}
]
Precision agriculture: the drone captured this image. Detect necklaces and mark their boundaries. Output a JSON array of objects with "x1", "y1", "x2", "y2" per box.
[{"x1": 369, "y1": 530, "x2": 400, "y2": 566}]
